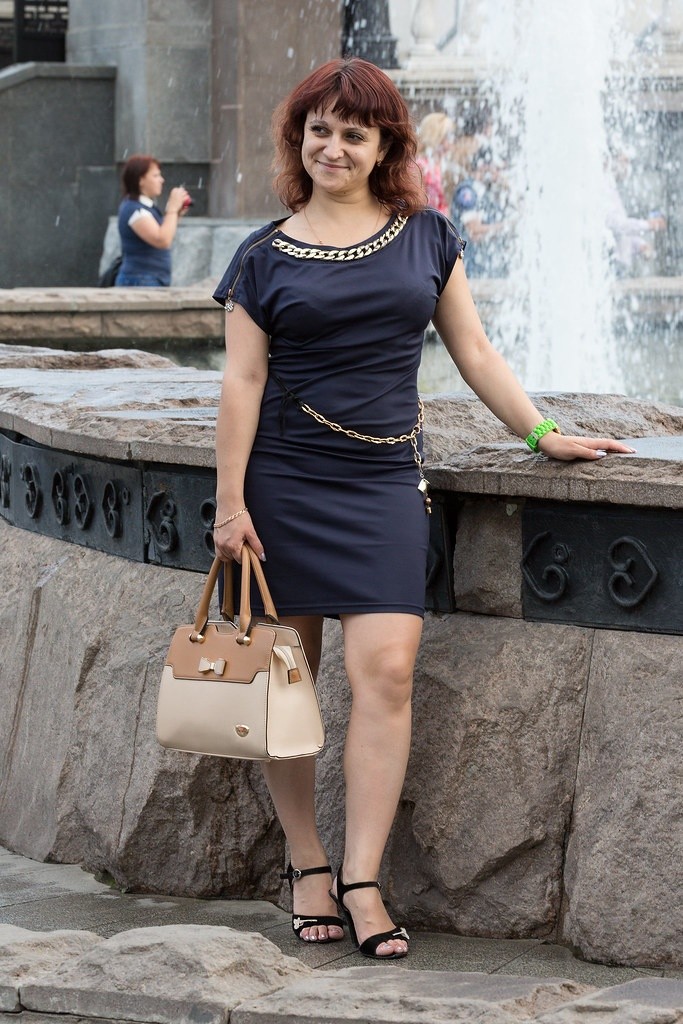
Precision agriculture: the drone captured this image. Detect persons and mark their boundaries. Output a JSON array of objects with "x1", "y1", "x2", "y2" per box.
[
  {"x1": 114, "y1": 155, "x2": 191, "y2": 286},
  {"x1": 210, "y1": 59, "x2": 638, "y2": 959},
  {"x1": 416, "y1": 112, "x2": 515, "y2": 276}
]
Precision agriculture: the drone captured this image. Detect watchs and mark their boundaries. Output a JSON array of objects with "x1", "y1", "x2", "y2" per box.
[{"x1": 526, "y1": 417, "x2": 561, "y2": 453}]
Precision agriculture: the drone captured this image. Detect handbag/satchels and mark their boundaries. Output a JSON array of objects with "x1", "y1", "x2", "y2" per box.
[{"x1": 155, "y1": 544, "x2": 326, "y2": 760}]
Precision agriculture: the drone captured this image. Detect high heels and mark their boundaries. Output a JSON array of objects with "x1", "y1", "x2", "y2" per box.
[
  {"x1": 282, "y1": 861, "x2": 347, "y2": 944},
  {"x1": 330, "y1": 865, "x2": 410, "y2": 958}
]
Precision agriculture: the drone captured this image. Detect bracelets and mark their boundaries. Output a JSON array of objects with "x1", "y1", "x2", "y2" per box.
[{"x1": 213, "y1": 509, "x2": 249, "y2": 529}]
